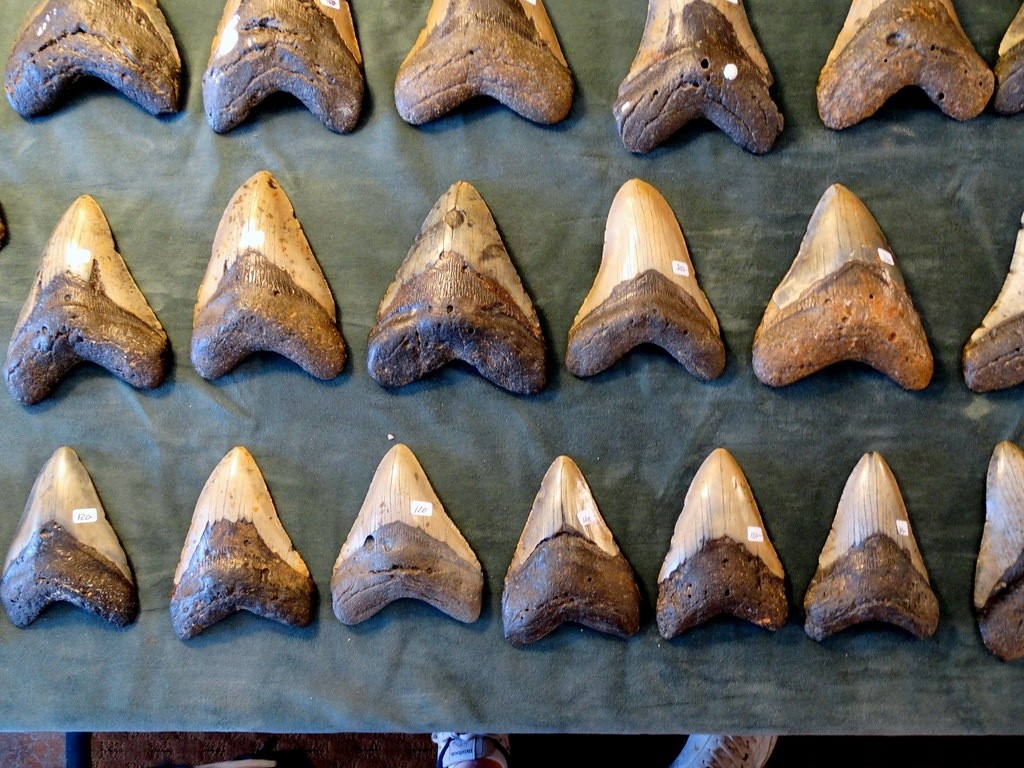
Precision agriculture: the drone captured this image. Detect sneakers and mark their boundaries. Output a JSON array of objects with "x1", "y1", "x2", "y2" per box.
[
  {"x1": 429, "y1": 732, "x2": 510, "y2": 768},
  {"x1": 672, "y1": 736, "x2": 776, "y2": 768}
]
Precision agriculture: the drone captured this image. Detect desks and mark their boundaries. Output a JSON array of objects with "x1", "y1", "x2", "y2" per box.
[{"x1": 0, "y1": 0, "x2": 1024, "y2": 736}]
